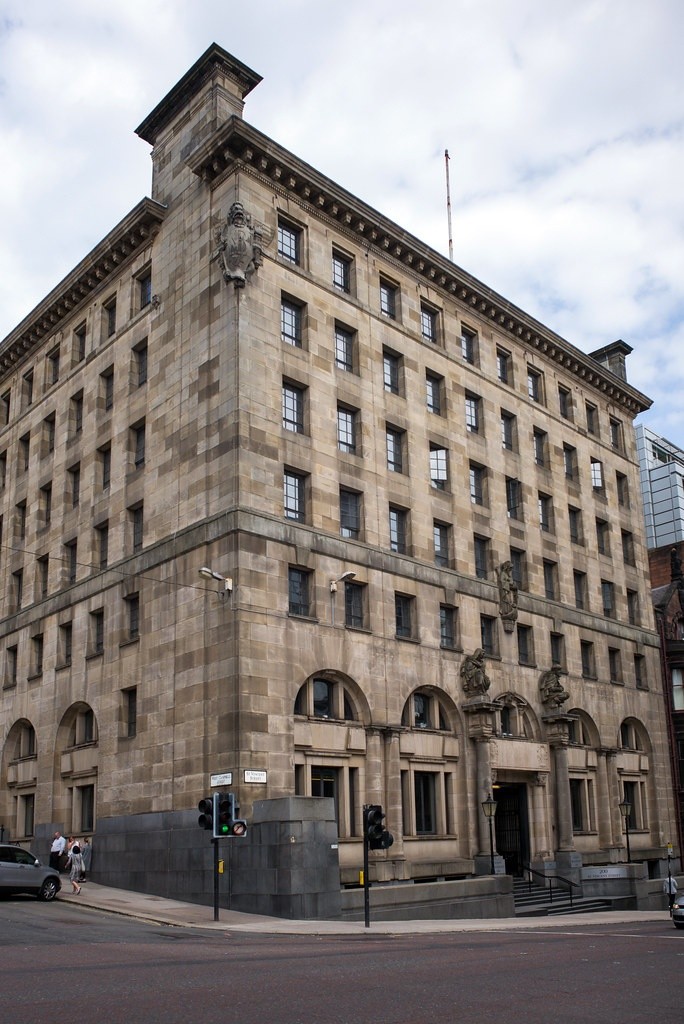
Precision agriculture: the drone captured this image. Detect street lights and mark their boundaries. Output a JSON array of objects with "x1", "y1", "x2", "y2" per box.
[
  {"x1": 481, "y1": 793, "x2": 498, "y2": 875},
  {"x1": 619, "y1": 799, "x2": 632, "y2": 863}
]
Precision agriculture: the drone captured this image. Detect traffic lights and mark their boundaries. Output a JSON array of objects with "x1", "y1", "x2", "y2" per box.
[
  {"x1": 363, "y1": 804, "x2": 394, "y2": 849},
  {"x1": 213, "y1": 792, "x2": 247, "y2": 838},
  {"x1": 197, "y1": 797, "x2": 215, "y2": 830}
]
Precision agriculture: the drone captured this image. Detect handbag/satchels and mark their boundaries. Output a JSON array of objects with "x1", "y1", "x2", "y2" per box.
[{"x1": 81, "y1": 860, "x2": 85, "y2": 871}]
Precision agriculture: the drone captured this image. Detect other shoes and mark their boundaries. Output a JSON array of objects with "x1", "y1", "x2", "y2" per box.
[{"x1": 77, "y1": 887, "x2": 81, "y2": 895}]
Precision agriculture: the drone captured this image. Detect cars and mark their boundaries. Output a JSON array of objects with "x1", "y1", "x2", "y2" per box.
[{"x1": 0, "y1": 844, "x2": 62, "y2": 901}]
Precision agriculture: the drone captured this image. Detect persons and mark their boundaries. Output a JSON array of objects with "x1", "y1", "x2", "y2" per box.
[
  {"x1": 64, "y1": 846, "x2": 81, "y2": 895},
  {"x1": 460, "y1": 648, "x2": 490, "y2": 690},
  {"x1": 498, "y1": 561, "x2": 518, "y2": 615},
  {"x1": 49, "y1": 832, "x2": 92, "y2": 883},
  {"x1": 540, "y1": 664, "x2": 570, "y2": 709},
  {"x1": 663, "y1": 873, "x2": 678, "y2": 911}
]
